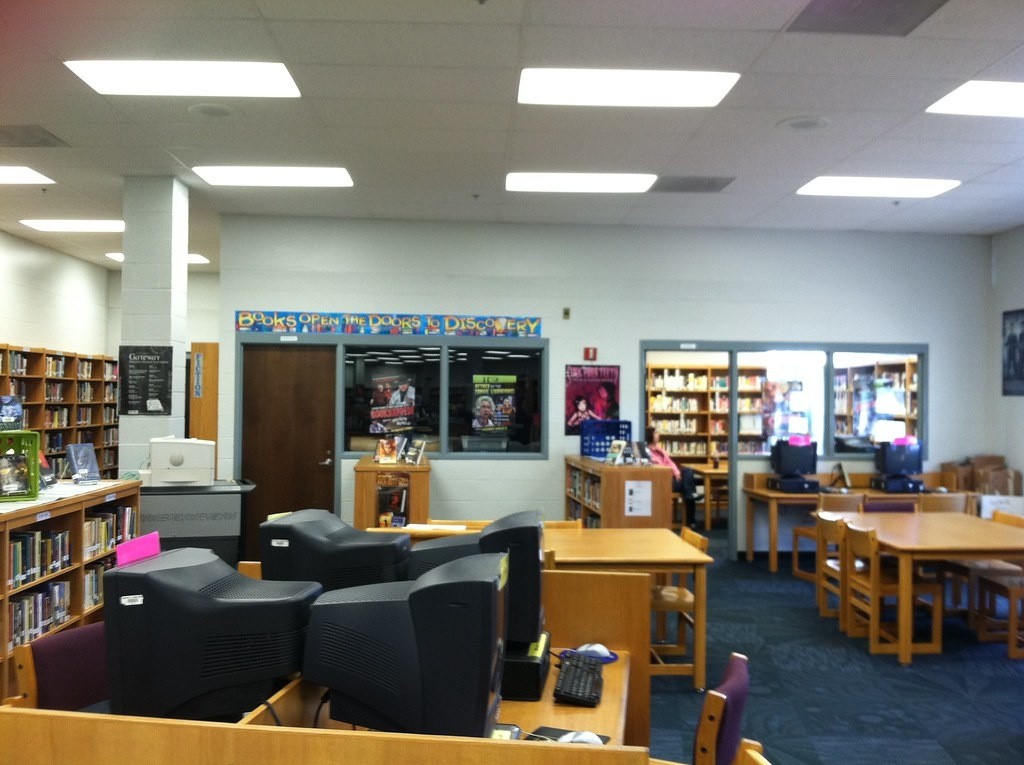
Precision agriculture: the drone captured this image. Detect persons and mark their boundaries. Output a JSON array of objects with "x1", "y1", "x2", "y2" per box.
[
  {"x1": 472, "y1": 396, "x2": 496, "y2": 427},
  {"x1": 646, "y1": 427, "x2": 704, "y2": 532},
  {"x1": 370, "y1": 375, "x2": 415, "y2": 433}
]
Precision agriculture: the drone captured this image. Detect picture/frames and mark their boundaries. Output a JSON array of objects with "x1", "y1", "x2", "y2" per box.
[{"x1": 1001, "y1": 308, "x2": 1024, "y2": 395}]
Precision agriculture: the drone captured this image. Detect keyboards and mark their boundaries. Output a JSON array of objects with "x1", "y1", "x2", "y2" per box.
[
  {"x1": 557, "y1": 732, "x2": 602, "y2": 746},
  {"x1": 553, "y1": 654, "x2": 604, "y2": 708}
]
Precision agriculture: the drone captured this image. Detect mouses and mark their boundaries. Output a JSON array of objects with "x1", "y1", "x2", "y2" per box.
[
  {"x1": 936, "y1": 487, "x2": 947, "y2": 494},
  {"x1": 838, "y1": 487, "x2": 847, "y2": 493},
  {"x1": 577, "y1": 644, "x2": 610, "y2": 658}
]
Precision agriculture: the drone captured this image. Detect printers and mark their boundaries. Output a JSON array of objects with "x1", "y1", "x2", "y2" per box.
[{"x1": 151, "y1": 435, "x2": 216, "y2": 487}]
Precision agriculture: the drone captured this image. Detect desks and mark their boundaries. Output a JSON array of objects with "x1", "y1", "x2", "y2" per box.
[
  {"x1": 814, "y1": 511, "x2": 1024, "y2": 646},
  {"x1": 744, "y1": 489, "x2": 980, "y2": 574},
  {"x1": 347, "y1": 650, "x2": 631, "y2": 747},
  {"x1": 681, "y1": 460, "x2": 730, "y2": 530},
  {"x1": 383, "y1": 528, "x2": 714, "y2": 694}
]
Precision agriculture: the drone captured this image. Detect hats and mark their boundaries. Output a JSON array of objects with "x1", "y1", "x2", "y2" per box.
[{"x1": 393, "y1": 375, "x2": 411, "y2": 385}]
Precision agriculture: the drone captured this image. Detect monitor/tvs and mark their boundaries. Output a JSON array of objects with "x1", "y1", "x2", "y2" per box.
[
  {"x1": 769, "y1": 440, "x2": 818, "y2": 479},
  {"x1": 101, "y1": 508, "x2": 544, "y2": 748},
  {"x1": 876, "y1": 441, "x2": 922, "y2": 480}
]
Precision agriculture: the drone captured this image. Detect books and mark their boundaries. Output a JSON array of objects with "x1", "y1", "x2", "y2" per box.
[
  {"x1": 837, "y1": 366, "x2": 921, "y2": 441},
  {"x1": 651, "y1": 369, "x2": 768, "y2": 452},
  {"x1": 376, "y1": 436, "x2": 424, "y2": 528},
  {"x1": 0, "y1": 349, "x2": 138, "y2": 651},
  {"x1": 568, "y1": 471, "x2": 602, "y2": 527}
]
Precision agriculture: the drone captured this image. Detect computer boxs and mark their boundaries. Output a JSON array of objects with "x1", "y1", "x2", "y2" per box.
[
  {"x1": 767, "y1": 477, "x2": 818, "y2": 494},
  {"x1": 500, "y1": 631, "x2": 551, "y2": 701},
  {"x1": 870, "y1": 479, "x2": 924, "y2": 493}
]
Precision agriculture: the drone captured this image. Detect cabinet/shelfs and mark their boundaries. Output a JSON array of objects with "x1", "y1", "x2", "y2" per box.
[
  {"x1": 835, "y1": 362, "x2": 918, "y2": 443},
  {"x1": 0, "y1": 344, "x2": 119, "y2": 479},
  {"x1": 1, "y1": 479, "x2": 144, "y2": 699},
  {"x1": 354, "y1": 452, "x2": 430, "y2": 526},
  {"x1": 647, "y1": 366, "x2": 768, "y2": 455},
  {"x1": 564, "y1": 452, "x2": 673, "y2": 529}
]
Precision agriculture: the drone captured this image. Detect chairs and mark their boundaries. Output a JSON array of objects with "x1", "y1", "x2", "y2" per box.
[
  {"x1": 0, "y1": 518, "x2": 777, "y2": 765},
  {"x1": 794, "y1": 487, "x2": 1024, "y2": 665}
]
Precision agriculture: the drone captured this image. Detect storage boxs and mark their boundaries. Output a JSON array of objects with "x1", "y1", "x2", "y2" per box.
[{"x1": 0, "y1": 429, "x2": 40, "y2": 503}]
